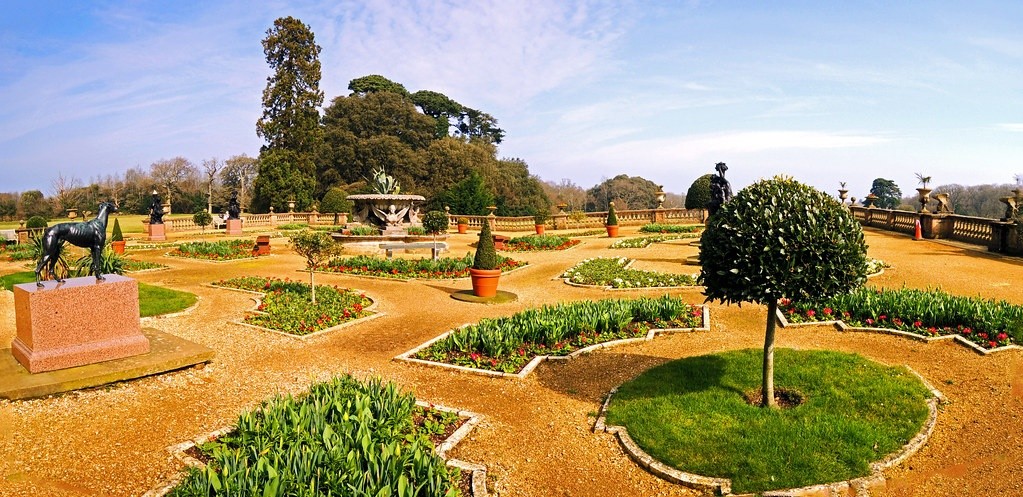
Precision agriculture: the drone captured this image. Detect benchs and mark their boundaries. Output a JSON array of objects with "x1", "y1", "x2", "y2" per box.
[
  {"x1": 477, "y1": 233, "x2": 510, "y2": 251},
  {"x1": 0, "y1": 229, "x2": 18, "y2": 252},
  {"x1": 379, "y1": 241, "x2": 446, "y2": 258},
  {"x1": 213, "y1": 215, "x2": 245, "y2": 230}
]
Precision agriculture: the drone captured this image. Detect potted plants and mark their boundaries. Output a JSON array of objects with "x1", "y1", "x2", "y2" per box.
[
  {"x1": 468, "y1": 217, "x2": 502, "y2": 298},
  {"x1": 534, "y1": 209, "x2": 552, "y2": 234},
  {"x1": 422, "y1": 210, "x2": 449, "y2": 236},
  {"x1": 407, "y1": 227, "x2": 422, "y2": 235},
  {"x1": 605, "y1": 204, "x2": 619, "y2": 237},
  {"x1": 458, "y1": 217, "x2": 468, "y2": 234},
  {"x1": 111, "y1": 218, "x2": 126, "y2": 254}
]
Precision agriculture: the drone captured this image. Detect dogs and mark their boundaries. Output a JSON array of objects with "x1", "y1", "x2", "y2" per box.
[{"x1": 34, "y1": 202, "x2": 119, "y2": 287}]
